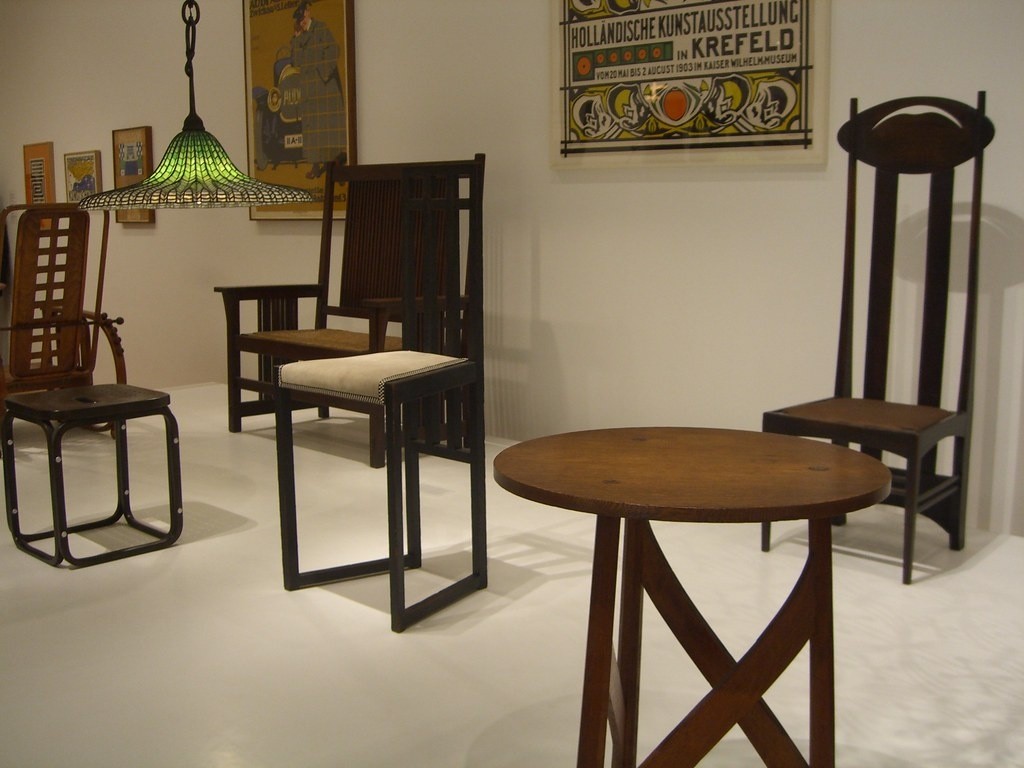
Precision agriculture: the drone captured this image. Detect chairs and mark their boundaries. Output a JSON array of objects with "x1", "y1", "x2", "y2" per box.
[
  {"x1": 214, "y1": 151, "x2": 487, "y2": 468},
  {"x1": 758, "y1": 87, "x2": 997, "y2": 585}
]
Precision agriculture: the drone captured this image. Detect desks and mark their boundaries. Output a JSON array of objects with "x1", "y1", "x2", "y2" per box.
[{"x1": 490, "y1": 425, "x2": 896, "y2": 768}]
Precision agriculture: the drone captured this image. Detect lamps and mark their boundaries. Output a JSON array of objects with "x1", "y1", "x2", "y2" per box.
[{"x1": 75, "y1": 0, "x2": 318, "y2": 210}]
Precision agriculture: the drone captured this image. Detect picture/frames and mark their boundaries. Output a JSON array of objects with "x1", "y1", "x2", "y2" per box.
[
  {"x1": 240, "y1": 0, "x2": 358, "y2": 223},
  {"x1": 111, "y1": 125, "x2": 155, "y2": 224},
  {"x1": 547, "y1": 1, "x2": 830, "y2": 176},
  {"x1": 23, "y1": 141, "x2": 57, "y2": 232},
  {"x1": 62, "y1": 149, "x2": 103, "y2": 204}
]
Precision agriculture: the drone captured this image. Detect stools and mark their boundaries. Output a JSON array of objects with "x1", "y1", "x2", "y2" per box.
[
  {"x1": 0, "y1": 382, "x2": 184, "y2": 568},
  {"x1": 273, "y1": 348, "x2": 489, "y2": 633}
]
